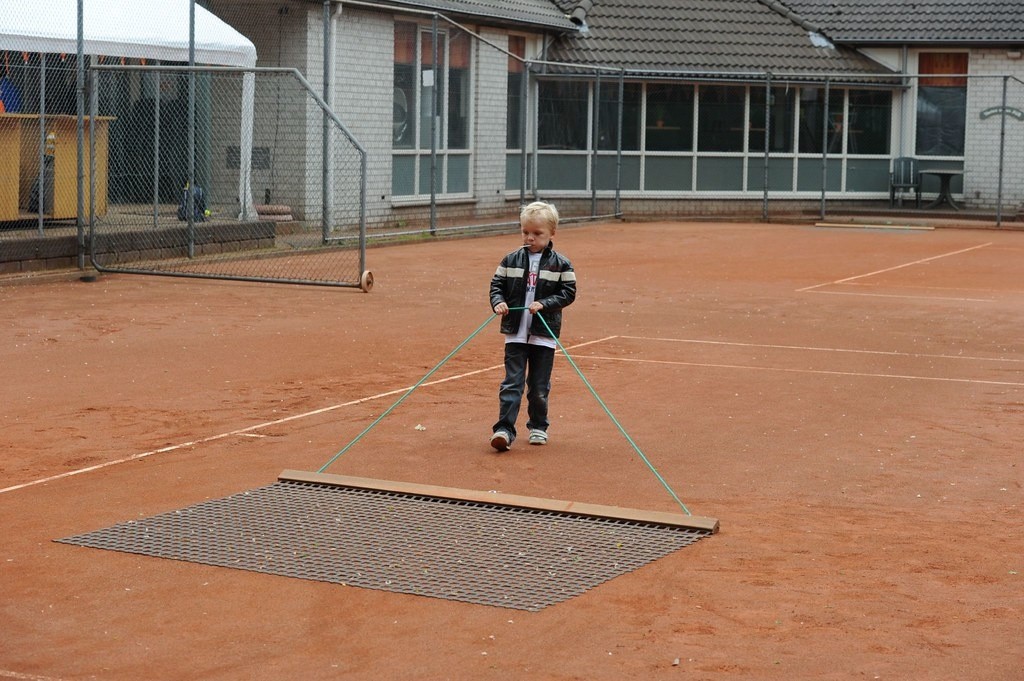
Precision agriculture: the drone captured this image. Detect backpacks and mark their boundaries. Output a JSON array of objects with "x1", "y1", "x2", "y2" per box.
[{"x1": 177, "y1": 183, "x2": 211, "y2": 223}]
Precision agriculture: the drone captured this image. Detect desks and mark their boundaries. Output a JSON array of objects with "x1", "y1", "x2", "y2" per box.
[{"x1": 917, "y1": 169, "x2": 970, "y2": 212}]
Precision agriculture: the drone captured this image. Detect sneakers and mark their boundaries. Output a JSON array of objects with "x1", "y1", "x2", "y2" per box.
[
  {"x1": 528, "y1": 428, "x2": 549, "y2": 445},
  {"x1": 490, "y1": 431, "x2": 511, "y2": 451}
]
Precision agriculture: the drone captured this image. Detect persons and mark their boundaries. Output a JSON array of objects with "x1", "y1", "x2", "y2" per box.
[{"x1": 489, "y1": 201, "x2": 577, "y2": 450}]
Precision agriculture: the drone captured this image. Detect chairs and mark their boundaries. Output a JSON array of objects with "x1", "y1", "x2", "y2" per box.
[{"x1": 888, "y1": 157, "x2": 924, "y2": 210}]
